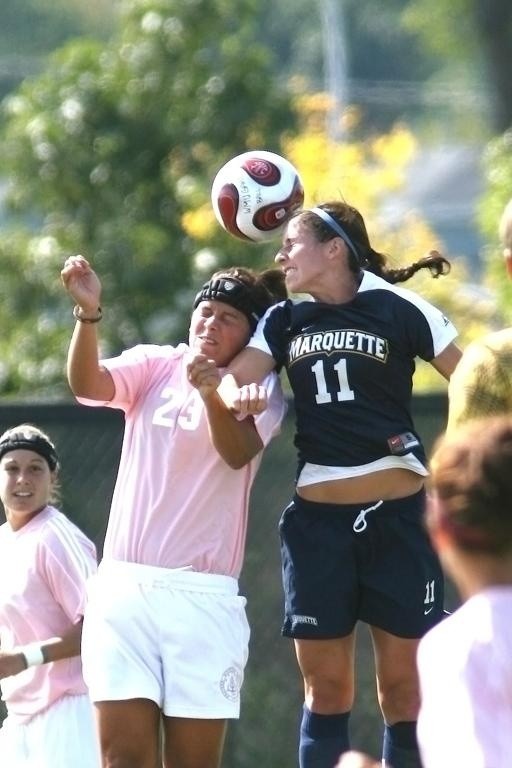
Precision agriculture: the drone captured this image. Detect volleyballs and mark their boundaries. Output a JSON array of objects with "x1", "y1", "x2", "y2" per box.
[{"x1": 212, "y1": 151, "x2": 303, "y2": 243}]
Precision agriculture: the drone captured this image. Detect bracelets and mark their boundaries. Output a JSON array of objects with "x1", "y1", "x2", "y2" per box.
[{"x1": 19, "y1": 641, "x2": 44, "y2": 668}]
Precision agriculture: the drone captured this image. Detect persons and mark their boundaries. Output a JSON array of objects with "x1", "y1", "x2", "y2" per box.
[
  {"x1": 57, "y1": 249, "x2": 288, "y2": 767},
  {"x1": 410, "y1": 414, "x2": 512, "y2": 768},
  {"x1": 0, "y1": 419, "x2": 103, "y2": 767},
  {"x1": 216, "y1": 196, "x2": 466, "y2": 767},
  {"x1": 443, "y1": 195, "x2": 512, "y2": 434}
]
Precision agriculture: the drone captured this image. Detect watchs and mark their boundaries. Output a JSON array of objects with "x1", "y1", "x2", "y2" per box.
[{"x1": 72, "y1": 301, "x2": 104, "y2": 324}]
